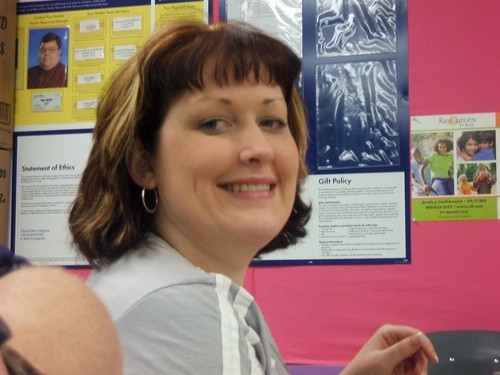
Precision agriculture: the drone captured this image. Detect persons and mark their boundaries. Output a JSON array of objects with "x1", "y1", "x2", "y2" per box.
[
  {"x1": 474, "y1": 163, "x2": 492, "y2": 185},
  {"x1": 28, "y1": 33, "x2": 67, "y2": 88},
  {"x1": 456, "y1": 131, "x2": 479, "y2": 161},
  {"x1": 419, "y1": 138, "x2": 455, "y2": 196},
  {"x1": 0, "y1": 247, "x2": 123, "y2": 375},
  {"x1": 411, "y1": 147, "x2": 431, "y2": 195},
  {"x1": 458, "y1": 130, "x2": 496, "y2": 161},
  {"x1": 458, "y1": 174, "x2": 478, "y2": 195},
  {"x1": 472, "y1": 171, "x2": 495, "y2": 194},
  {"x1": 68, "y1": 17, "x2": 440, "y2": 375}
]
